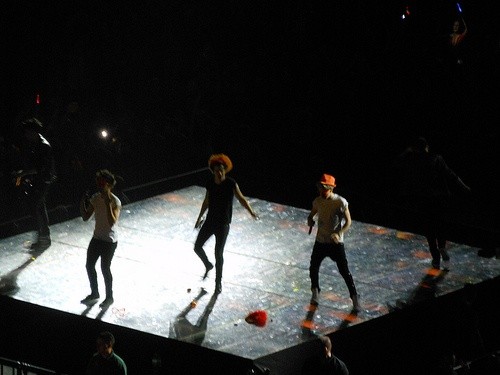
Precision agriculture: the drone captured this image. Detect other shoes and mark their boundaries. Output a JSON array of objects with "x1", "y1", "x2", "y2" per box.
[
  {"x1": 100, "y1": 297, "x2": 113, "y2": 307},
  {"x1": 353, "y1": 302, "x2": 361, "y2": 314},
  {"x1": 441, "y1": 248, "x2": 450, "y2": 261},
  {"x1": 85, "y1": 293, "x2": 99, "y2": 301},
  {"x1": 433, "y1": 254, "x2": 440, "y2": 268},
  {"x1": 311, "y1": 297, "x2": 319, "y2": 306}
]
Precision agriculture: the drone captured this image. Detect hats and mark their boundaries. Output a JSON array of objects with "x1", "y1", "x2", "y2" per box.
[
  {"x1": 96, "y1": 169, "x2": 116, "y2": 186},
  {"x1": 22, "y1": 118, "x2": 43, "y2": 132},
  {"x1": 320, "y1": 173, "x2": 337, "y2": 188}
]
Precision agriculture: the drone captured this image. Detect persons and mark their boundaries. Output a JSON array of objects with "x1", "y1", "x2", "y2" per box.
[
  {"x1": 299, "y1": 335, "x2": 349, "y2": 375},
  {"x1": 80, "y1": 171, "x2": 122, "y2": 304},
  {"x1": 21, "y1": 116, "x2": 55, "y2": 248},
  {"x1": 448, "y1": 17, "x2": 467, "y2": 48},
  {"x1": 306, "y1": 173, "x2": 361, "y2": 313},
  {"x1": 417, "y1": 146, "x2": 459, "y2": 270},
  {"x1": 86, "y1": 332, "x2": 127, "y2": 375},
  {"x1": 195, "y1": 154, "x2": 255, "y2": 295}
]
